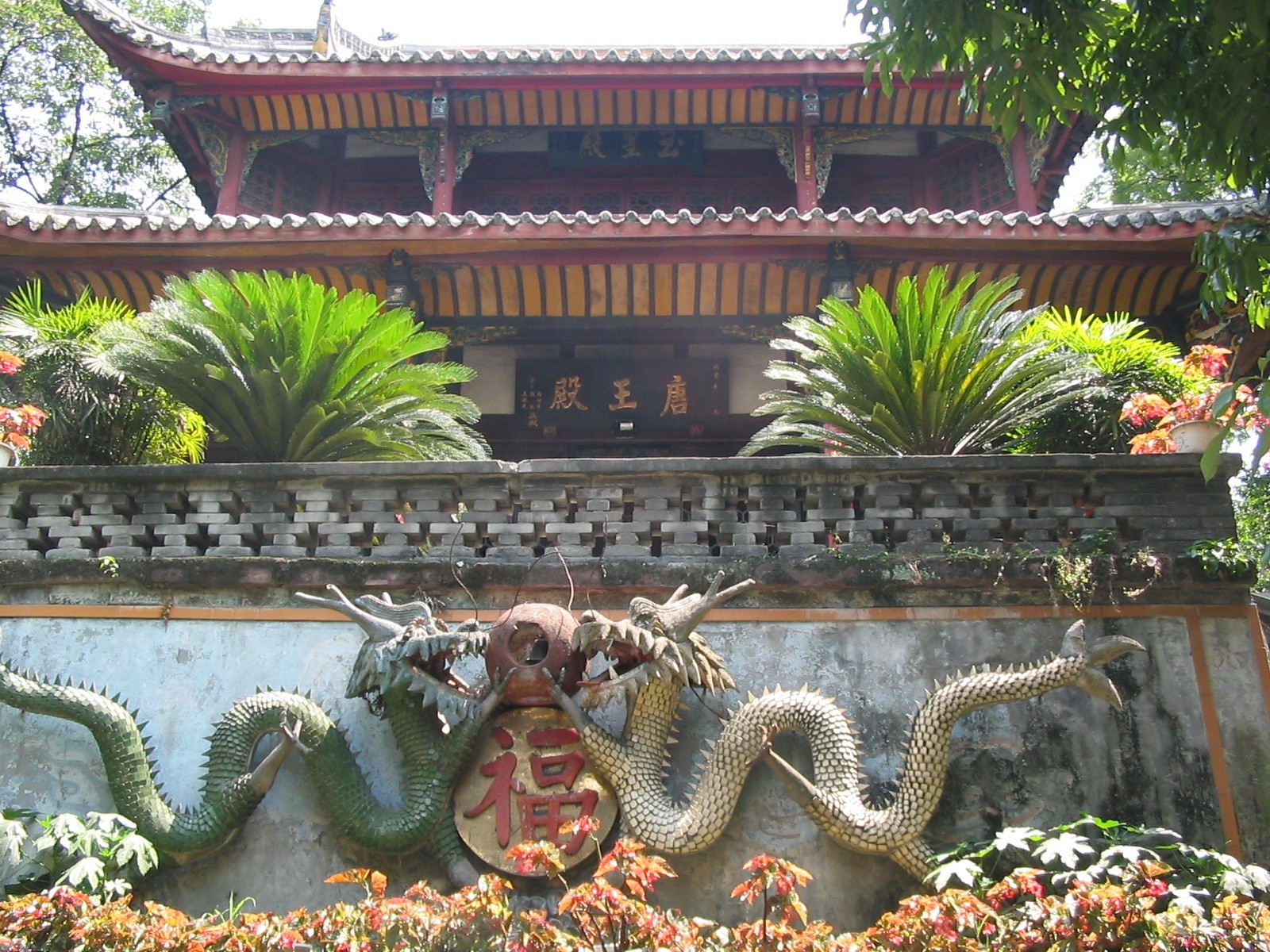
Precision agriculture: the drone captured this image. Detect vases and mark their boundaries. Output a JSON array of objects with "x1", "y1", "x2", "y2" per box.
[
  {"x1": 1169, "y1": 420, "x2": 1222, "y2": 456},
  {"x1": 0, "y1": 443, "x2": 16, "y2": 468}
]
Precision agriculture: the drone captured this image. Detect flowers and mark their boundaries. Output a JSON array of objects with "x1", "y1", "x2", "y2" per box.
[
  {"x1": 0, "y1": 350, "x2": 49, "y2": 458},
  {"x1": 1117, "y1": 344, "x2": 1270, "y2": 455}
]
